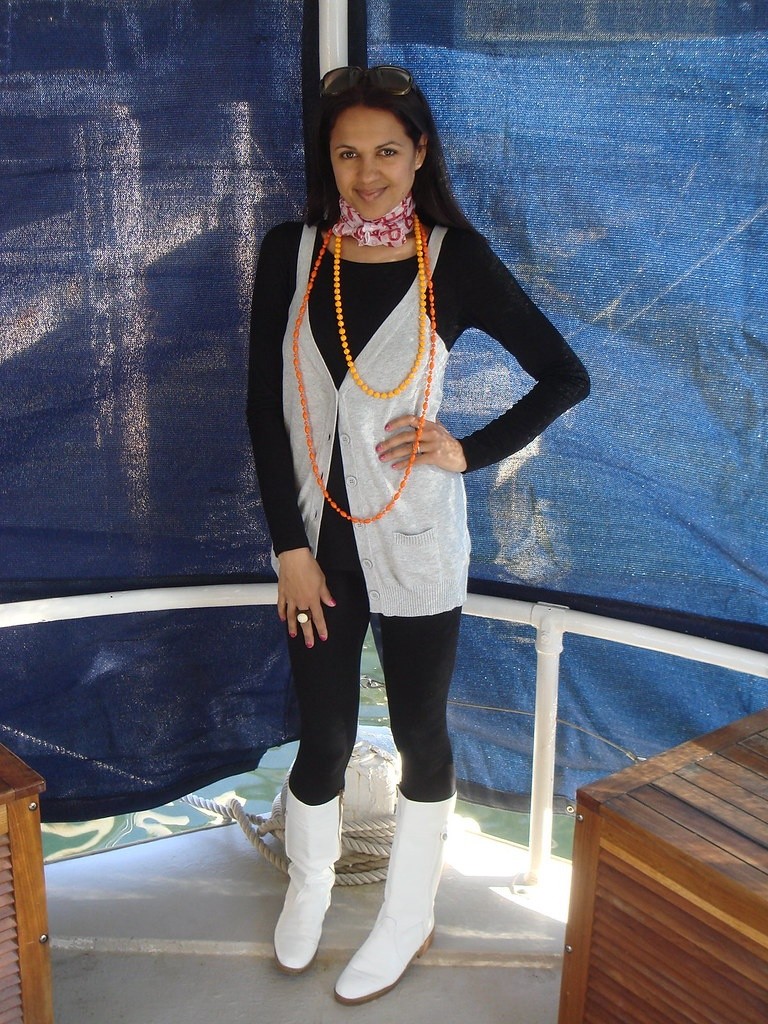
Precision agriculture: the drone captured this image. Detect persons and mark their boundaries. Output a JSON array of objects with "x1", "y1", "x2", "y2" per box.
[{"x1": 246, "y1": 65, "x2": 590, "y2": 1006}]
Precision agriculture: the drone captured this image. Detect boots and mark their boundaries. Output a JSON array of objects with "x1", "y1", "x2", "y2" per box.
[
  {"x1": 273, "y1": 784, "x2": 345, "y2": 975},
  {"x1": 334, "y1": 781, "x2": 457, "y2": 1005}
]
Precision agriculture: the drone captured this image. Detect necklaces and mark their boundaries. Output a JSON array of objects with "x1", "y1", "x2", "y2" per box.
[
  {"x1": 293, "y1": 221, "x2": 436, "y2": 523},
  {"x1": 334, "y1": 217, "x2": 426, "y2": 398}
]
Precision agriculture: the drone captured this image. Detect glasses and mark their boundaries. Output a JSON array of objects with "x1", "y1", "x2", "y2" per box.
[{"x1": 318, "y1": 65, "x2": 418, "y2": 97}]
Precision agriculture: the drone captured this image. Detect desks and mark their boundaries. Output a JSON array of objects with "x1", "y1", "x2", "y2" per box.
[
  {"x1": 1, "y1": 743, "x2": 53, "y2": 1024},
  {"x1": 559, "y1": 708, "x2": 768, "y2": 1024}
]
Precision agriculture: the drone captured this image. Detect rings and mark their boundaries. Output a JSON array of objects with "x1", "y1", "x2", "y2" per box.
[
  {"x1": 297, "y1": 610, "x2": 312, "y2": 624},
  {"x1": 413, "y1": 443, "x2": 420, "y2": 454}
]
